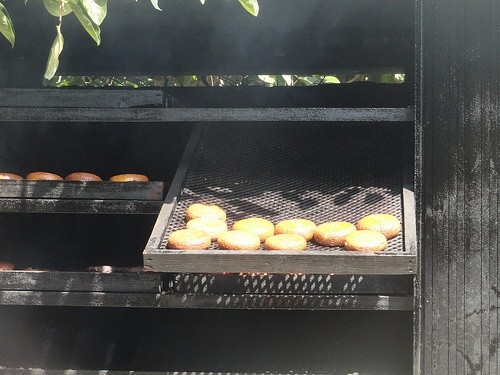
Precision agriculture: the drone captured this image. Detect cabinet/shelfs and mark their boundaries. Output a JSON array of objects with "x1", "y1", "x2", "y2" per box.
[{"x1": 0, "y1": 1, "x2": 500, "y2": 375}]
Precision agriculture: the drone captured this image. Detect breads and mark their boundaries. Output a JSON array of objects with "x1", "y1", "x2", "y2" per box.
[
  {"x1": 167, "y1": 202, "x2": 400, "y2": 252},
  {"x1": 0, "y1": 170, "x2": 150, "y2": 182},
  {"x1": 0, "y1": 259, "x2": 61, "y2": 271}
]
